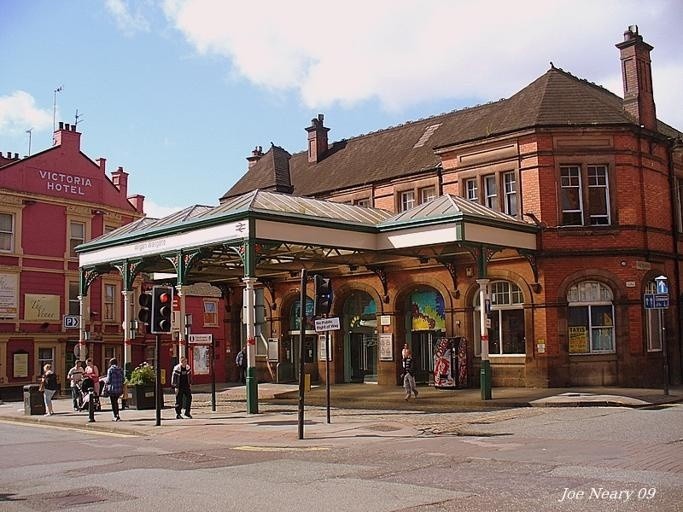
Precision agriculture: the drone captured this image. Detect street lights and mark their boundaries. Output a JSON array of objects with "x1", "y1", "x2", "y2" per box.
[{"x1": 184, "y1": 312, "x2": 193, "y2": 358}]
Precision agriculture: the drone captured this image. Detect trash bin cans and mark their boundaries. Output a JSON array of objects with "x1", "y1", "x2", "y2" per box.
[{"x1": 23, "y1": 383, "x2": 46, "y2": 416}]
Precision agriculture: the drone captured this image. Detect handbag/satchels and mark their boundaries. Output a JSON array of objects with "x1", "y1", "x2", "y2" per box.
[
  {"x1": 81, "y1": 378, "x2": 94, "y2": 392},
  {"x1": 45, "y1": 374, "x2": 57, "y2": 390},
  {"x1": 100, "y1": 384, "x2": 109, "y2": 398}
]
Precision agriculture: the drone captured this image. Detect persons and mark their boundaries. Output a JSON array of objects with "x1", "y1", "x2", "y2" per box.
[
  {"x1": 171, "y1": 356, "x2": 195, "y2": 420},
  {"x1": 66, "y1": 357, "x2": 101, "y2": 411},
  {"x1": 104, "y1": 358, "x2": 123, "y2": 422},
  {"x1": 400, "y1": 344, "x2": 409, "y2": 368},
  {"x1": 401, "y1": 349, "x2": 418, "y2": 400},
  {"x1": 235, "y1": 344, "x2": 248, "y2": 383},
  {"x1": 39, "y1": 363, "x2": 57, "y2": 417}
]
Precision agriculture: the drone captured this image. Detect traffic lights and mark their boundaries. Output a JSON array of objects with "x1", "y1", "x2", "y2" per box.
[
  {"x1": 151, "y1": 285, "x2": 172, "y2": 335},
  {"x1": 314, "y1": 275, "x2": 332, "y2": 316},
  {"x1": 138, "y1": 290, "x2": 152, "y2": 322}
]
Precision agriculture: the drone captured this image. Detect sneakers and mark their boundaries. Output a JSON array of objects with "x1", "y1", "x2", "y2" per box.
[
  {"x1": 44, "y1": 411, "x2": 54, "y2": 417},
  {"x1": 112, "y1": 417, "x2": 121, "y2": 422},
  {"x1": 404, "y1": 391, "x2": 418, "y2": 401},
  {"x1": 176, "y1": 412, "x2": 193, "y2": 420}
]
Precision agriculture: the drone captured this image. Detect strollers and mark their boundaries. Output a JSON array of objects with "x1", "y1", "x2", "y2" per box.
[{"x1": 76, "y1": 378, "x2": 100, "y2": 411}]
